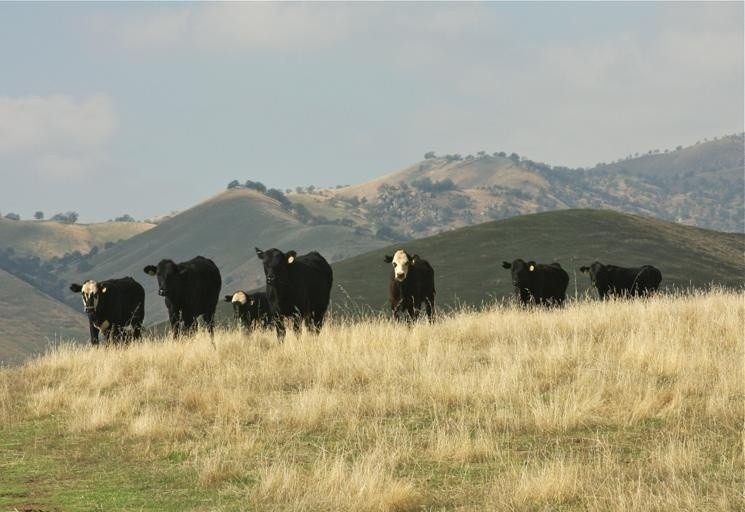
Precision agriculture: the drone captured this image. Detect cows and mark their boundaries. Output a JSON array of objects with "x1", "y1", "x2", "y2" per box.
[
  {"x1": 71, "y1": 276, "x2": 145, "y2": 349},
  {"x1": 226, "y1": 291, "x2": 272, "y2": 333},
  {"x1": 255, "y1": 248, "x2": 333, "y2": 345},
  {"x1": 144, "y1": 256, "x2": 221, "y2": 350},
  {"x1": 385, "y1": 248, "x2": 434, "y2": 326},
  {"x1": 502, "y1": 258, "x2": 569, "y2": 310},
  {"x1": 580, "y1": 261, "x2": 662, "y2": 303}
]
